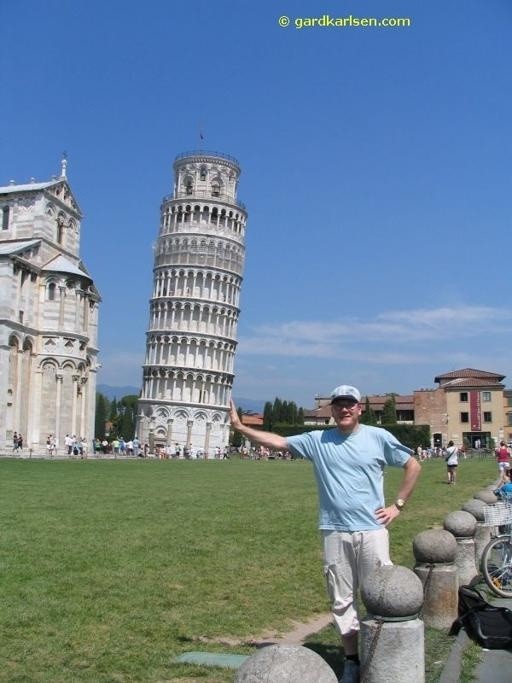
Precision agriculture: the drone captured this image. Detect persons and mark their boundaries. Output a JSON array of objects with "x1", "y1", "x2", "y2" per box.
[
  {"x1": 444, "y1": 440, "x2": 458, "y2": 485},
  {"x1": 12, "y1": 431, "x2": 481, "y2": 462},
  {"x1": 494, "y1": 440, "x2": 512, "y2": 479},
  {"x1": 227, "y1": 384, "x2": 422, "y2": 682}
]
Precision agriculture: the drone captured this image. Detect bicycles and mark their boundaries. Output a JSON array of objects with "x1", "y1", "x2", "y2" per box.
[{"x1": 480, "y1": 483, "x2": 512, "y2": 600}]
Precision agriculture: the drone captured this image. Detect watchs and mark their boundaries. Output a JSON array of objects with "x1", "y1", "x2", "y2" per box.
[{"x1": 394, "y1": 496, "x2": 406, "y2": 510}]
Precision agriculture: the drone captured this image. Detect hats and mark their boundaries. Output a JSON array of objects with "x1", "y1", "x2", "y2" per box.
[{"x1": 330, "y1": 384, "x2": 362, "y2": 402}]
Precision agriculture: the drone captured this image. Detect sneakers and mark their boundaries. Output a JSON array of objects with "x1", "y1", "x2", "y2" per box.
[{"x1": 338, "y1": 658, "x2": 361, "y2": 683}]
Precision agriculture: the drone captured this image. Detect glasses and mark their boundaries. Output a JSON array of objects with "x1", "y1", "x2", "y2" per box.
[{"x1": 331, "y1": 402, "x2": 358, "y2": 409}]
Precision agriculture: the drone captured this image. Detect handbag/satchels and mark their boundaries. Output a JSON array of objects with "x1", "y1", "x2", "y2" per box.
[
  {"x1": 447, "y1": 584, "x2": 512, "y2": 656},
  {"x1": 445, "y1": 447, "x2": 456, "y2": 462}
]
